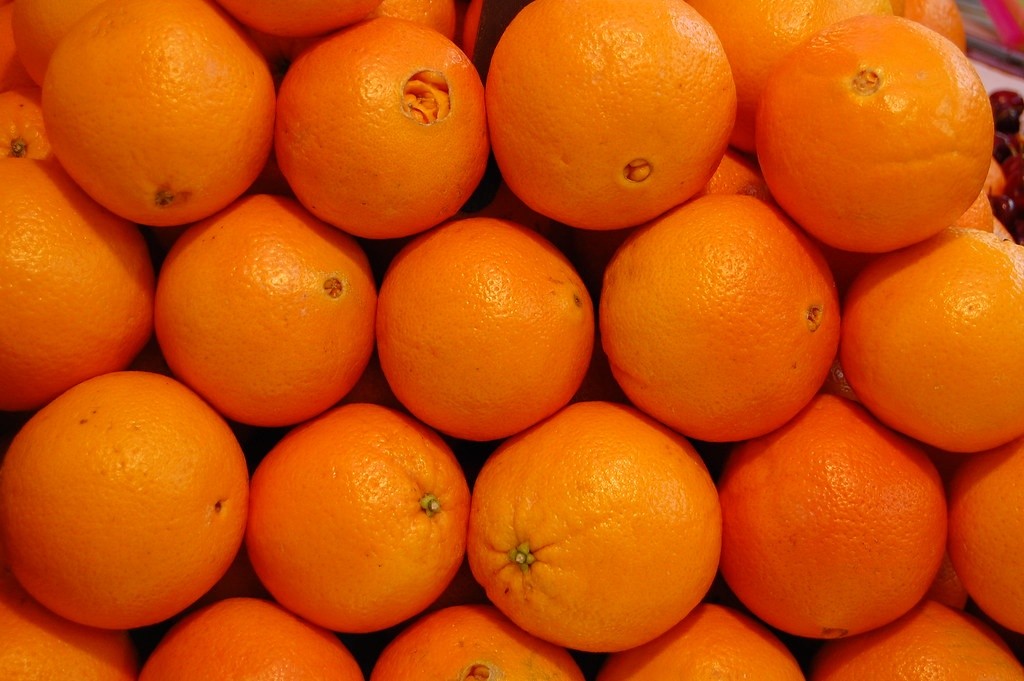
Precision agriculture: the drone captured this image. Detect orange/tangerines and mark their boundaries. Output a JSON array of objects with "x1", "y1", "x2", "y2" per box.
[{"x1": 0, "y1": 0, "x2": 1024, "y2": 681}]
